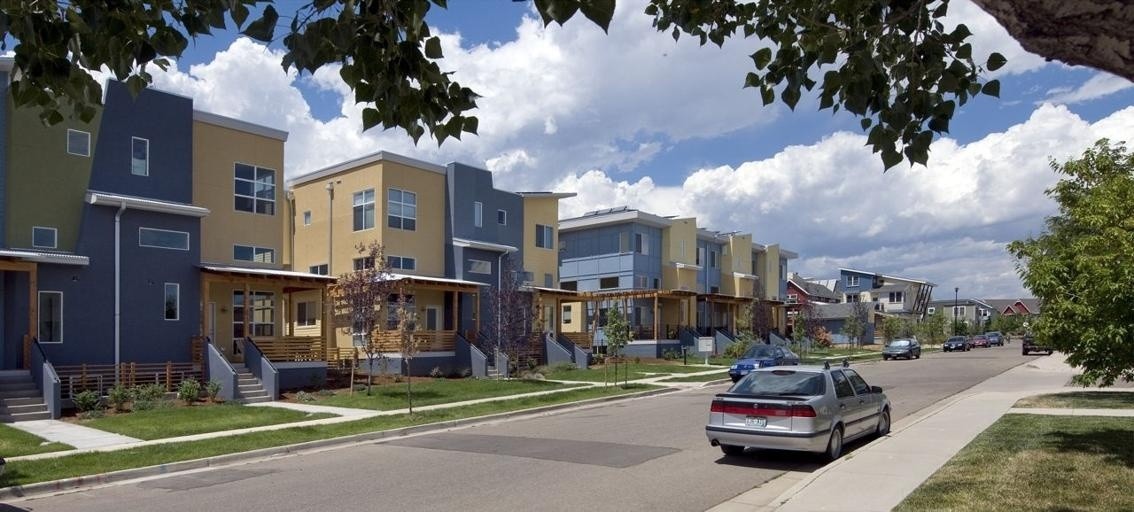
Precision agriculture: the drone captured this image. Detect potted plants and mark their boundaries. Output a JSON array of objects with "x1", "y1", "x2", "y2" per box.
[{"x1": 71, "y1": 377, "x2": 222, "y2": 415}]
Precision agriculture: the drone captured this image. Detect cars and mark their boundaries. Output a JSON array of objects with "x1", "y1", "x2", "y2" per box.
[
  {"x1": 705, "y1": 364, "x2": 892, "y2": 464},
  {"x1": 1019, "y1": 326, "x2": 1053, "y2": 355},
  {"x1": 944, "y1": 336, "x2": 971, "y2": 352},
  {"x1": 968, "y1": 335, "x2": 991, "y2": 348},
  {"x1": 881, "y1": 338, "x2": 921, "y2": 360},
  {"x1": 727, "y1": 344, "x2": 800, "y2": 382},
  {"x1": 985, "y1": 332, "x2": 1004, "y2": 346}
]
